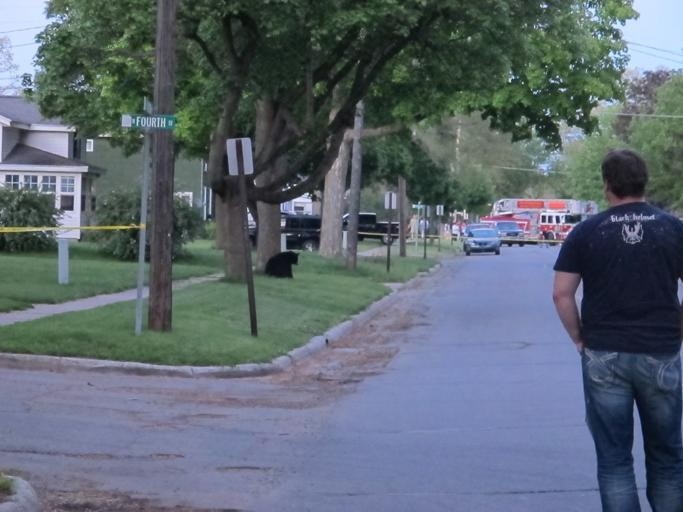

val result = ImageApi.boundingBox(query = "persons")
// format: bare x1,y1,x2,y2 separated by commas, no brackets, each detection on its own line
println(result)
419,219,429,238
445,220,466,242
553,149,682,512
408,213,419,240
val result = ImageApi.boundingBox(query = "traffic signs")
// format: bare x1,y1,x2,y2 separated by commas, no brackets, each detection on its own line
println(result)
121,114,176,129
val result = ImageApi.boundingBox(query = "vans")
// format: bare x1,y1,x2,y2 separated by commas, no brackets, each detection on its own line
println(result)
247,213,321,253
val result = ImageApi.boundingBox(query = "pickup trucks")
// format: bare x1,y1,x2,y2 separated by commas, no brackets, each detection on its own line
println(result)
342,212,399,245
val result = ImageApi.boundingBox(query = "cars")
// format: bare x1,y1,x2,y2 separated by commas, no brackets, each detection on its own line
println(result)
411,217,524,257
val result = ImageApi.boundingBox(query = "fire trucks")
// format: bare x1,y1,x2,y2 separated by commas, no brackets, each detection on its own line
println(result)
480,212,588,247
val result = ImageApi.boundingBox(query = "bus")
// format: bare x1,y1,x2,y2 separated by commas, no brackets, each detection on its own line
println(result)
491,199,597,248
